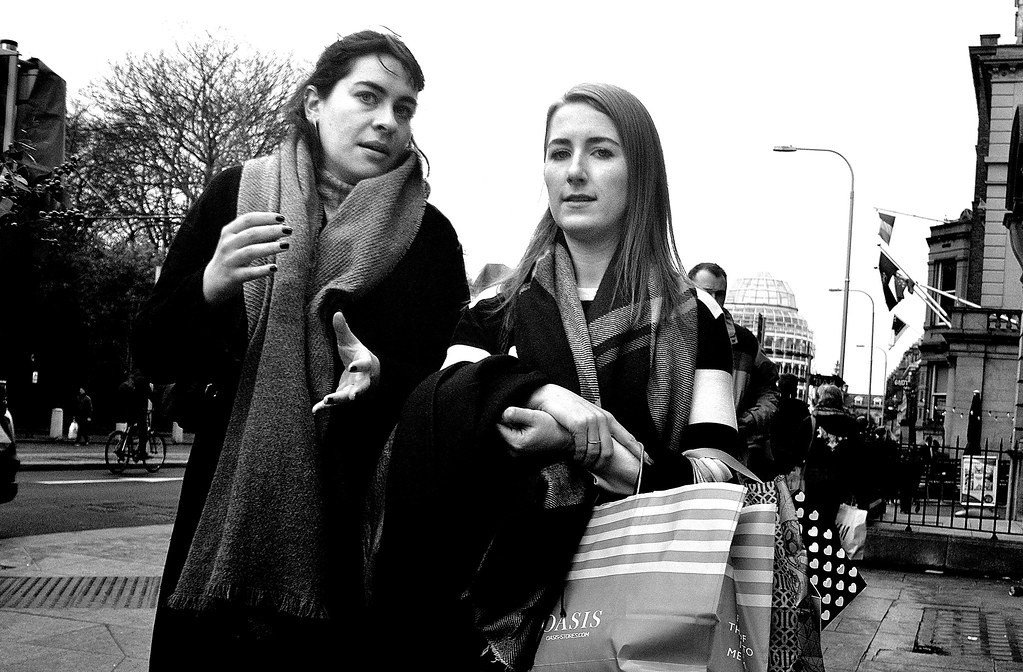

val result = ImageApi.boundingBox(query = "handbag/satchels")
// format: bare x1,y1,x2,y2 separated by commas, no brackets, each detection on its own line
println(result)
68,418,78,439
531,441,868,672
834,496,867,560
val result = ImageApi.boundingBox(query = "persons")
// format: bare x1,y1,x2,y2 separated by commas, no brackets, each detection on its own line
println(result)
685,263,783,468
409,81,738,672
126,375,155,459
75,386,93,445
131,31,471,672
772,371,897,523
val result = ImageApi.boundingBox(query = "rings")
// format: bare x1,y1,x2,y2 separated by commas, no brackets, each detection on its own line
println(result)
587,439,601,445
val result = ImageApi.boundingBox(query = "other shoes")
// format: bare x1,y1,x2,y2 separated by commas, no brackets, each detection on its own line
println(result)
137,453,150,460
114,450,124,460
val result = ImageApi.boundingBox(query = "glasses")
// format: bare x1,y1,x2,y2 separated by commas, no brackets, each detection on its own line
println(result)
705,289,726,299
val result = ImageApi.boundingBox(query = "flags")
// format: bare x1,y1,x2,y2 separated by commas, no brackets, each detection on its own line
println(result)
877,254,899,311
887,316,909,351
905,278,915,294
878,212,895,243
893,272,908,302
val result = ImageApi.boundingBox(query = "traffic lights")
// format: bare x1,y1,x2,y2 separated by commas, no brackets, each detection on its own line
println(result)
15,57,67,182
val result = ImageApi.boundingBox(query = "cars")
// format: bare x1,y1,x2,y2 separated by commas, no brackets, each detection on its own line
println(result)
0,380,20,504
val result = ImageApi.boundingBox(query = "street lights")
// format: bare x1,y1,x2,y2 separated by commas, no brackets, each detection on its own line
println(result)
856,345,887,426
773,146,854,379
829,289,874,428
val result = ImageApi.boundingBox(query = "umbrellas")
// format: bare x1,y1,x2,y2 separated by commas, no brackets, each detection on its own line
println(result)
962,389,983,456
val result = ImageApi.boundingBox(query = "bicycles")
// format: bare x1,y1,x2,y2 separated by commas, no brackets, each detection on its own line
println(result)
105,408,166,474
